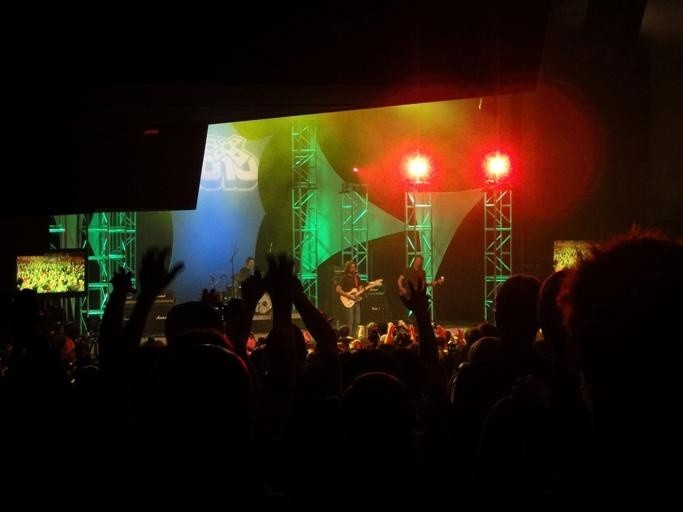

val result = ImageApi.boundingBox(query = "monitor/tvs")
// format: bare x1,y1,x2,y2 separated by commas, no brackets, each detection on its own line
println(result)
12,248,88,298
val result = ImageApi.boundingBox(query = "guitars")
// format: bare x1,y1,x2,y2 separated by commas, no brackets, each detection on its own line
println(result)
340,279,384,309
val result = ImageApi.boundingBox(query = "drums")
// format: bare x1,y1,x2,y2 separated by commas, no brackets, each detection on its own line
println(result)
254,293,271,315
227,286,242,299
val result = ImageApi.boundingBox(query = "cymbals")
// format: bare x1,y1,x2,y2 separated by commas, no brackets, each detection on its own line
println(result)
230,273,251,279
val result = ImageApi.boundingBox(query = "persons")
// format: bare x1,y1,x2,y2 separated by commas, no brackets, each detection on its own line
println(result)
0,222,683,512
